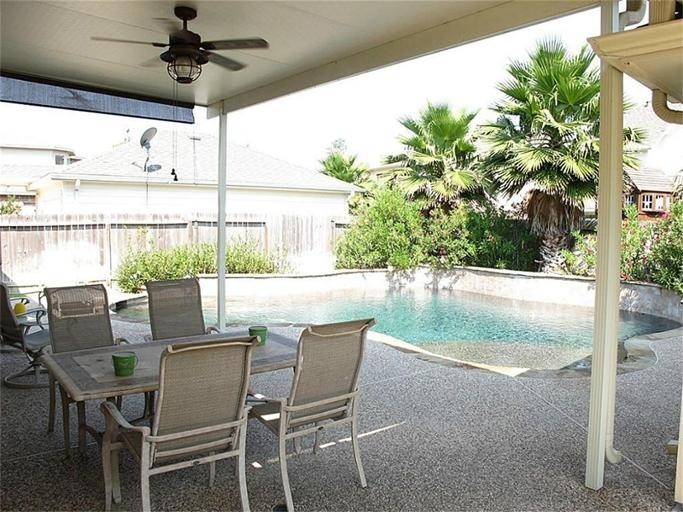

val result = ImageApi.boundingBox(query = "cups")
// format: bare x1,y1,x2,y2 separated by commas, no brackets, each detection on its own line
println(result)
249,326,267,345
111,352,139,376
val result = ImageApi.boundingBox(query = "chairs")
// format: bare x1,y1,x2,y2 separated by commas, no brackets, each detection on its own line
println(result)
144,276,222,347
42,283,131,457
1,280,60,390
231,317,378,512
99,334,261,512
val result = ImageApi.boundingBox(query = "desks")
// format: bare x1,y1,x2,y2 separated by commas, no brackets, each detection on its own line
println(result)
40,327,301,505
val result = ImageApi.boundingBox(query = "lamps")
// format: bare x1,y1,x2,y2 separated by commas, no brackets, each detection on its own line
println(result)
160,4,209,85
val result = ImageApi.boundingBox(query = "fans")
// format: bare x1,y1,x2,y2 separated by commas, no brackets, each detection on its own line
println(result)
90,4,270,72
